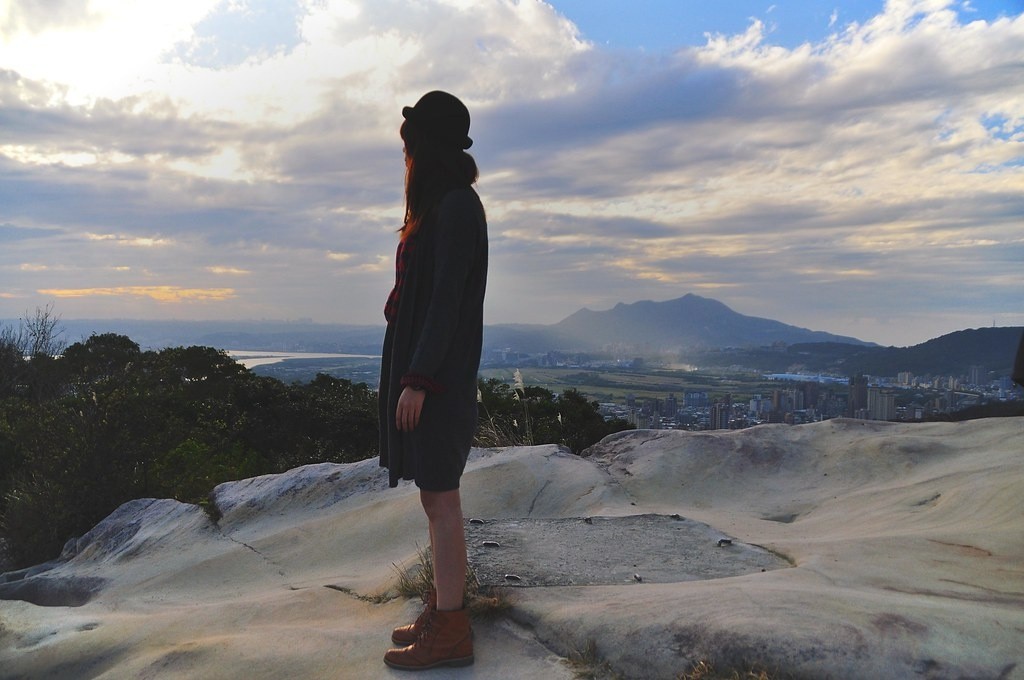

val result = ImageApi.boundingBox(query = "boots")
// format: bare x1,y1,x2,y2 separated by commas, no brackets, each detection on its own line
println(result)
383,588,475,671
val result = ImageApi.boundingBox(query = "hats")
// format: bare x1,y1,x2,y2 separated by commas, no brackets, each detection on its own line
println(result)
402,91,473,149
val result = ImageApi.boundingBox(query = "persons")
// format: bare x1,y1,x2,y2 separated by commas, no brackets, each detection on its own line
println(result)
377,91,488,671
